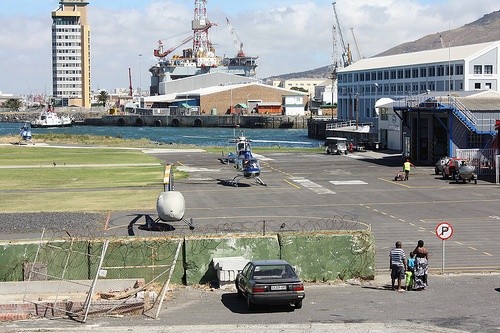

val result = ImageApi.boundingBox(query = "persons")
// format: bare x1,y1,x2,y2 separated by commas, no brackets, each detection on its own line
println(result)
413,240,429,288
407,251,415,269
389,241,407,291
403,159,414,181
404,266,414,291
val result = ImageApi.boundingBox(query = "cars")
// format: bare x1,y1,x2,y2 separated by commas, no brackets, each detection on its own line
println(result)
435,157,478,184
235,260,305,311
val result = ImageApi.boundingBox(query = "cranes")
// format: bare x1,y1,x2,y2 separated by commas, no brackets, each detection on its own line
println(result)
154,0,258,66
331,1,363,77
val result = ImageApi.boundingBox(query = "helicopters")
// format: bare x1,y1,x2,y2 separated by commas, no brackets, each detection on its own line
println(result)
18,120,35,145
219,130,267,187
147,163,195,230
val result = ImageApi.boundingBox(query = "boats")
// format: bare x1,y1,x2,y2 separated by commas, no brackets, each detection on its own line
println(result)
30,104,73,128
